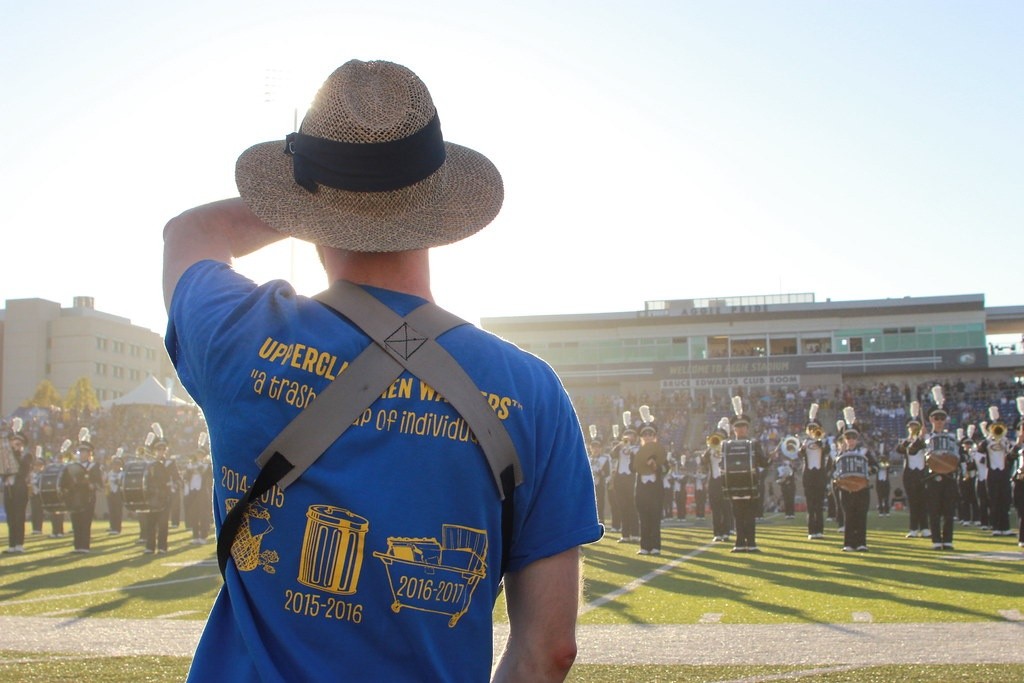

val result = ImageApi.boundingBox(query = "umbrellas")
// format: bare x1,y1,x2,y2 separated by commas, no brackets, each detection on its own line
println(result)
101,375,189,406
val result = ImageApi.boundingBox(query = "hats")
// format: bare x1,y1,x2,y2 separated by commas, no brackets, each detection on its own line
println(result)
730,415,751,426
961,436,974,445
833,433,844,444
621,425,638,436
590,437,602,445
610,437,622,445
841,424,860,434
638,422,657,435
153,438,169,447
714,428,728,440
35,458,45,465
197,446,209,454
1013,416,1024,430
805,418,821,429
925,404,949,421
113,457,125,470
236,61,502,253
905,417,923,428
78,441,94,451
10,430,28,445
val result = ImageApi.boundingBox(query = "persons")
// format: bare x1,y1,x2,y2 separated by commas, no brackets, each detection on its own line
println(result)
577,377,1024,554
711,345,766,372
806,345,820,354
0,404,216,554
162,58,604,683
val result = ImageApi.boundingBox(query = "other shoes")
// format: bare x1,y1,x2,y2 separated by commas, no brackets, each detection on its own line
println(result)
71,548,90,554
637,548,660,555
661,518,686,523
933,543,953,551
144,550,153,554
982,525,993,530
610,528,622,533
32,531,42,534
907,529,932,538
191,537,206,545
958,520,981,527
808,533,823,539
879,514,891,518
843,546,868,551
714,535,730,541
158,549,167,556
838,527,845,533
617,536,639,544
992,530,1016,536
109,531,118,535
730,547,757,552
826,517,833,522
136,538,145,543
785,515,795,519
696,517,705,520
4,545,24,553
170,525,177,528
729,530,735,536
1019,542,1024,547
49,534,63,538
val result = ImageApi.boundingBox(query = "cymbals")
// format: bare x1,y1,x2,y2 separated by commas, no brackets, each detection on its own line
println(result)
632,441,667,476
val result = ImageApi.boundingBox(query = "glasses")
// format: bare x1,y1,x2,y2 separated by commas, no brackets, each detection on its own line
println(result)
845,434,857,440
933,415,947,421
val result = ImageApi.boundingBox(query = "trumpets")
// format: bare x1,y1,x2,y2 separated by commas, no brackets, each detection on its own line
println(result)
704,431,725,451
808,425,827,442
990,421,1009,442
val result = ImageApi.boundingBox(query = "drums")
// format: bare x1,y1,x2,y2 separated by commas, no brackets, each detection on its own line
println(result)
36,450,156,511
923,432,965,475
831,451,871,495
718,436,763,512
